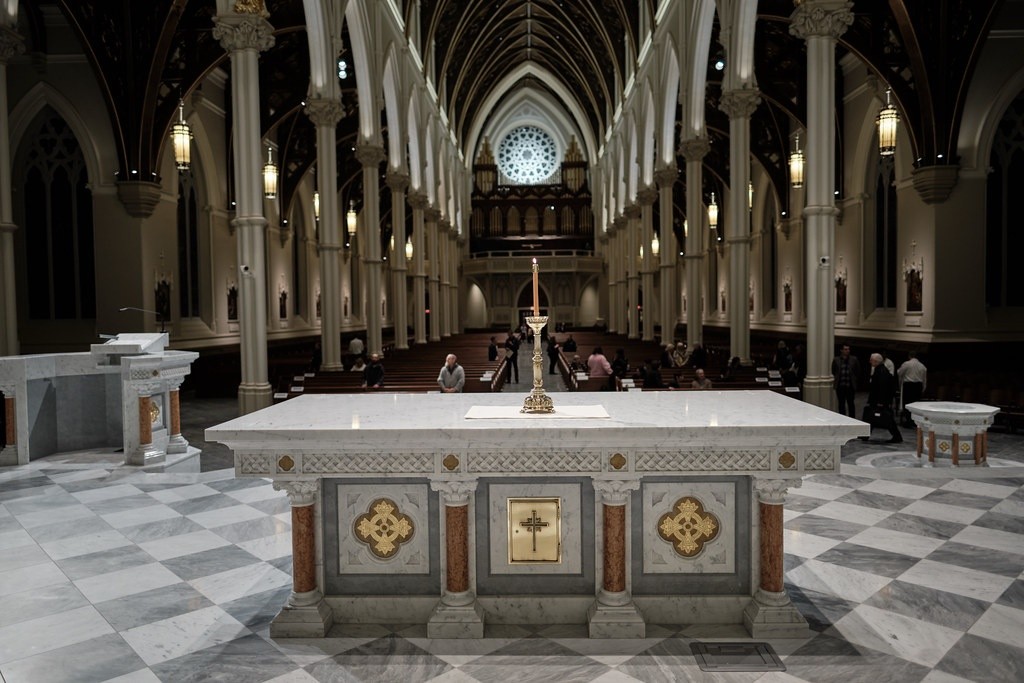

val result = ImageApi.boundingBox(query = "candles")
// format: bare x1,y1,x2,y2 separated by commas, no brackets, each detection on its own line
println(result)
532,257,539,316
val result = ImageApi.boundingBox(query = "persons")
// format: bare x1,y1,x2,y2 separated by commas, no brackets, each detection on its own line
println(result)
546,336,560,376
570,346,630,391
351,353,385,388
437,354,465,392
563,334,577,352
520,323,547,345
773,340,807,401
633,342,746,390
488,336,499,361
831,342,862,419
856,351,926,444
505,330,520,385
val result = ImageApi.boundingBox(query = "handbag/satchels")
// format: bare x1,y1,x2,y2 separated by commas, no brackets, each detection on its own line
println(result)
863,401,894,429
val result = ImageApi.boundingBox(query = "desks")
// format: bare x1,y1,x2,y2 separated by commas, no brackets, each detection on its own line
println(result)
906,402,1000,466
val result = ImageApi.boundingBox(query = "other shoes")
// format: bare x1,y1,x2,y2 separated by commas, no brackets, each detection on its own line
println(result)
857,437,869,441
549,373,556,374
506,382,511,384
516,381,518,383
885,436,903,443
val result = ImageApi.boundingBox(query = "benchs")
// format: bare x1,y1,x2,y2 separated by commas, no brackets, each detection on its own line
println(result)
547,330,799,399
273,333,521,393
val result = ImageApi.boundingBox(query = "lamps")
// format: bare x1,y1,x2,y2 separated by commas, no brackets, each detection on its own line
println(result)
406,235,414,262
347,200,357,237
875,87,899,156
707,191,718,229
639,244,644,260
651,231,659,256
683,219,688,236
749,181,753,212
788,132,805,189
313,192,319,222
262,132,279,199
167,100,195,170
